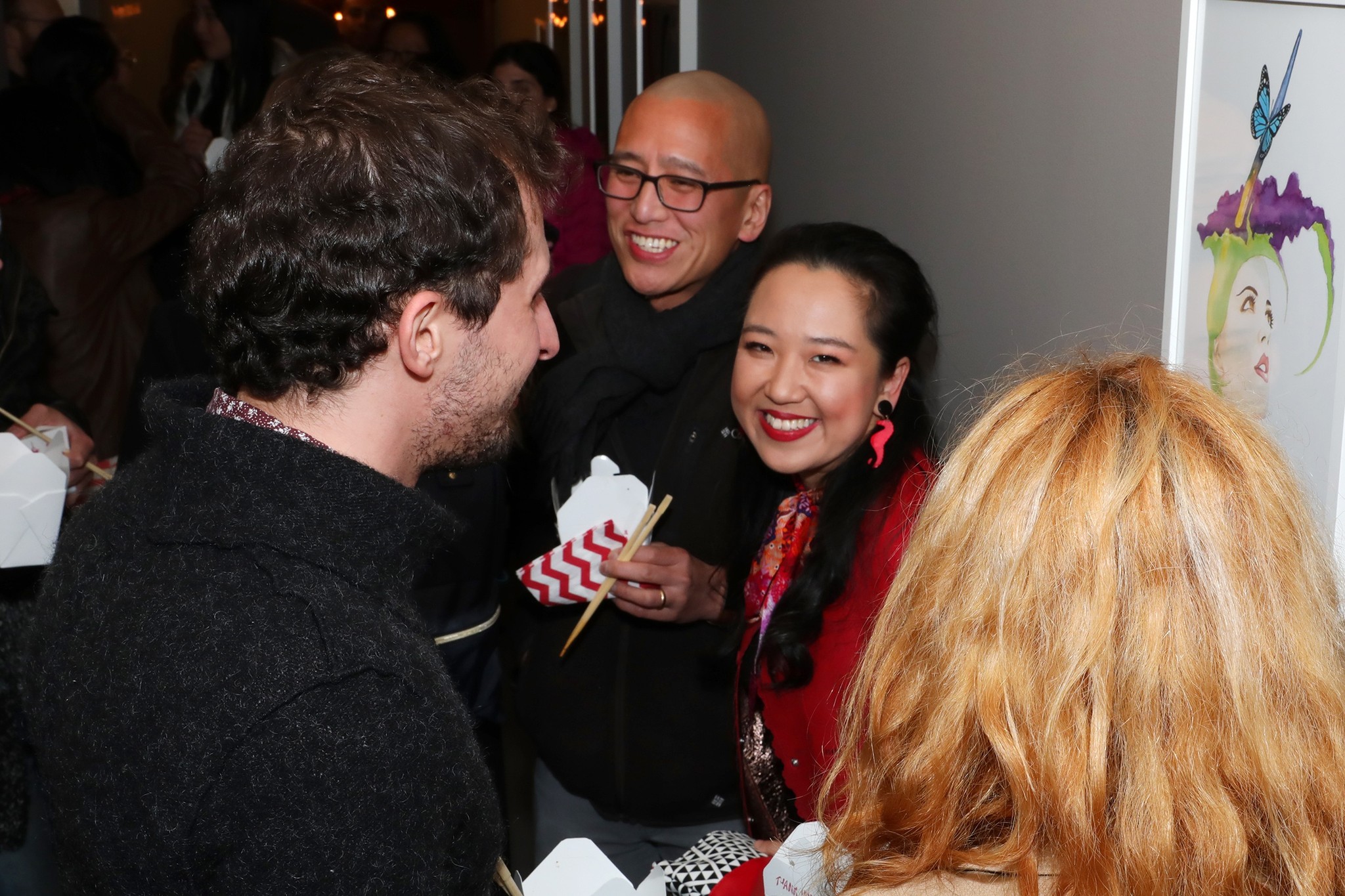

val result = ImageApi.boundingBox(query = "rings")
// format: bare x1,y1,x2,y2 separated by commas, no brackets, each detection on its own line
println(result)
660,589,666,607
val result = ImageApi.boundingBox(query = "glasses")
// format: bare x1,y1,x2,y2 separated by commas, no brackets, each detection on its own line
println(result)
382,48,423,65
593,158,763,214
117,50,138,68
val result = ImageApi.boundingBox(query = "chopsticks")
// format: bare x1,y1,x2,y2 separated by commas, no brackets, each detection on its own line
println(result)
560,493,673,657
494,855,522,896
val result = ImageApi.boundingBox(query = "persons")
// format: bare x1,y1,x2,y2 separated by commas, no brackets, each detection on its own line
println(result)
0,220,94,603
481,41,610,276
721,222,939,842
0,0,25,76
0,15,144,196
157,0,273,162
327,0,417,68
0,91,209,458
412,68,773,834
0,47,561,896
376,13,446,69
711,344,1345,896
